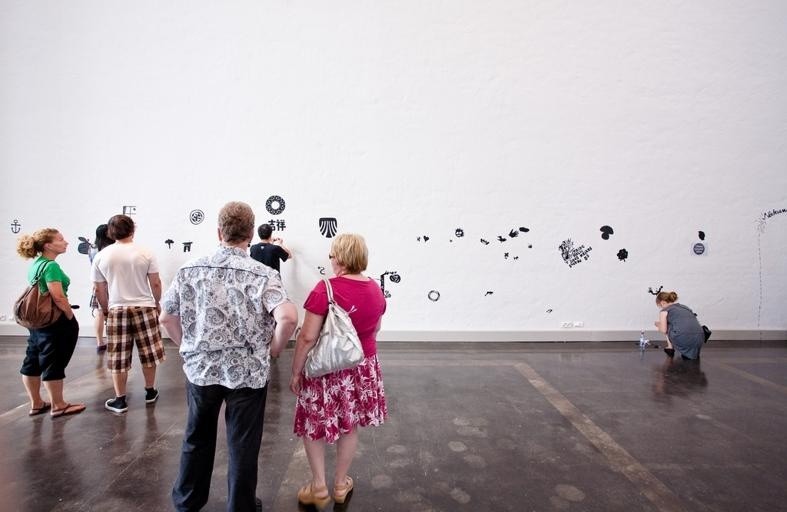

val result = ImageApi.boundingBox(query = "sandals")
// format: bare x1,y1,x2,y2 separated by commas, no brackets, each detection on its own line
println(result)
330,475,353,504
296,481,334,512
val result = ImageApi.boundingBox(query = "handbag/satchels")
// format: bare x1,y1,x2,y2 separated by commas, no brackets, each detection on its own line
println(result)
13,282,63,330
303,303,366,380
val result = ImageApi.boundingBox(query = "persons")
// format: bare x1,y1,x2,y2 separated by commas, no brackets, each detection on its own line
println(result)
288,233,387,510
87,224,114,354
653,290,704,359
156,200,298,511
90,214,162,414
18,227,86,417
251,223,291,317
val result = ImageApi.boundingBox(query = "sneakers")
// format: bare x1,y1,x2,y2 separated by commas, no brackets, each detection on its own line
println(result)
104,398,128,412
28,399,51,415
50,401,86,417
144,387,159,403
96,340,107,352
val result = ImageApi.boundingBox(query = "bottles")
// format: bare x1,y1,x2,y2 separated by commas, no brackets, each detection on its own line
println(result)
640,331,646,350
640,351,646,365
